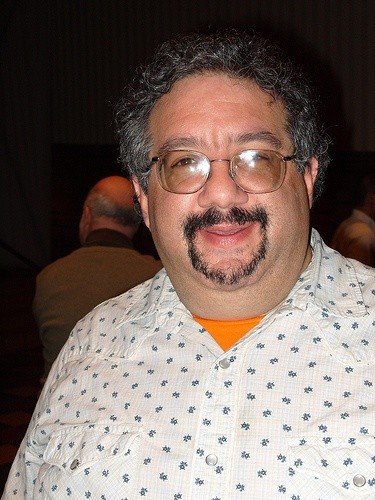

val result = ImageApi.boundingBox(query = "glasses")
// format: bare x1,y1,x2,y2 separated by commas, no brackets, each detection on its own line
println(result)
149,148,294,195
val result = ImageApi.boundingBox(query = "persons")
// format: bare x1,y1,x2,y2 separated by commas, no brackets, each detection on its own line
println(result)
29,176,164,384
0,40,375,499
329,166,375,270
